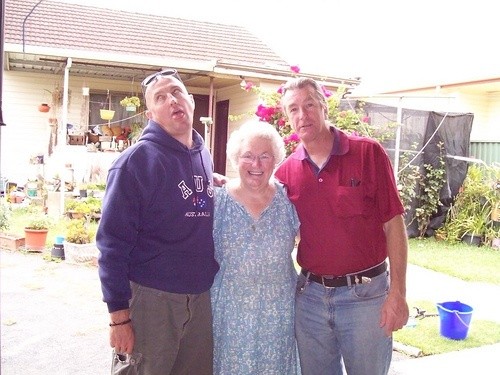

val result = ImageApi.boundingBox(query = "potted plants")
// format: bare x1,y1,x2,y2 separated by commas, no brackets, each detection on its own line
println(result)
0,161,112,263
434,159,500,250
120,95,142,112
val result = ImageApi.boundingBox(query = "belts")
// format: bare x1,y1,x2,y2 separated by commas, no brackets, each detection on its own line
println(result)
301,262,386,289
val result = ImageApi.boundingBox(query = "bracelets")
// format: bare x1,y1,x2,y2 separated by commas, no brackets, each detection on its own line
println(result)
109,319,131,327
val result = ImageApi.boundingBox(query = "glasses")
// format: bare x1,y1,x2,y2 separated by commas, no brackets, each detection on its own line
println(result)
239,152,274,166
141,69,182,96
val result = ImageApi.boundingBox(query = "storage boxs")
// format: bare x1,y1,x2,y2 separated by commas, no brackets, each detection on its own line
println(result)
100,108,115,120
68,134,85,145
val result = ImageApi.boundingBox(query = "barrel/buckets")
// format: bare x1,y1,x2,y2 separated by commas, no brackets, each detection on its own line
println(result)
100,95,115,120
436,301,473,341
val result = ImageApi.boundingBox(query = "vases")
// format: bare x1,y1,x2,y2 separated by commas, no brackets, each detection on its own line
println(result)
37,103,51,113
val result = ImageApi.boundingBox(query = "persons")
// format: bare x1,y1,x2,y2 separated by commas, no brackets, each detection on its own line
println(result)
212,76,409,375
96,76,219,375
210,121,301,375
